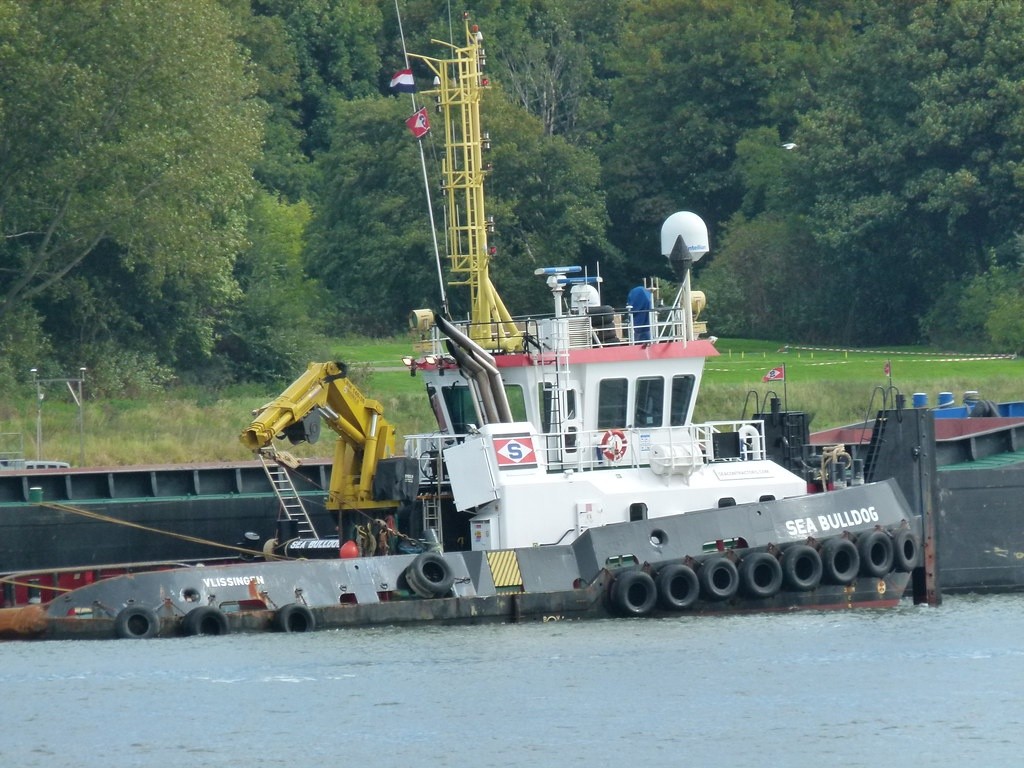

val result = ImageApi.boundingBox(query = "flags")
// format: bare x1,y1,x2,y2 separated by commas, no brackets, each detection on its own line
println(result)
762,365,786,383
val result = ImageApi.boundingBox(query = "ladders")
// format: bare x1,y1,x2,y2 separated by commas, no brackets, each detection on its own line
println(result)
256,441,320,542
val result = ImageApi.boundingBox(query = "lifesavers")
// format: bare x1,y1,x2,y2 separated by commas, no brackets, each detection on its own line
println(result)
182,606,230,637
893,532,919,572
609,571,657,617
397,552,454,598
856,529,895,577
820,537,861,585
781,545,822,590
654,563,701,611
740,552,783,598
116,605,161,638
276,603,314,633
699,558,740,599
602,431,627,460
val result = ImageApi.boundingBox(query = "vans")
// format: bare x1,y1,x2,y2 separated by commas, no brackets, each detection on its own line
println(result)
23,461,71,470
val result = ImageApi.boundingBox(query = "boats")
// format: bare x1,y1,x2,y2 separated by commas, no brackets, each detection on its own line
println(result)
0,390,1023,594
0,0,922,643
